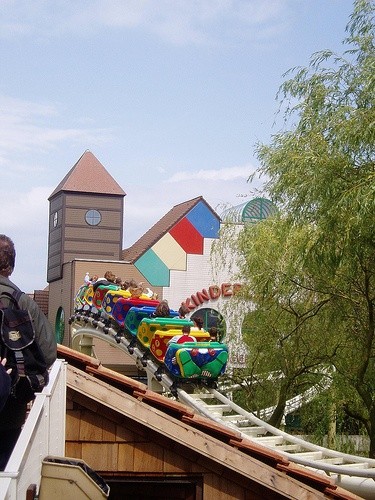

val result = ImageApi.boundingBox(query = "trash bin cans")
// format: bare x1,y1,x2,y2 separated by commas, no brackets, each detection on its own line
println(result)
37,456,111,500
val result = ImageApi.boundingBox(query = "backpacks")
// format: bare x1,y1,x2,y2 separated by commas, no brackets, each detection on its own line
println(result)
0,290,49,402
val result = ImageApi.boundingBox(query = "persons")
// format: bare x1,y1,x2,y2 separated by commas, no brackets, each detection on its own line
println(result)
148,293,159,302
84,271,153,298
127,289,142,302
166,325,197,347
0,234,57,471
149,302,170,319
200,327,222,344
172,307,189,319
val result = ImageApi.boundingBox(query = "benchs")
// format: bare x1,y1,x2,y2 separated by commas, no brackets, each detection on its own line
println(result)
164,339,229,379
114,298,161,324
124,307,181,334
91,284,119,307
149,329,209,362
137,318,195,347
101,291,153,316
